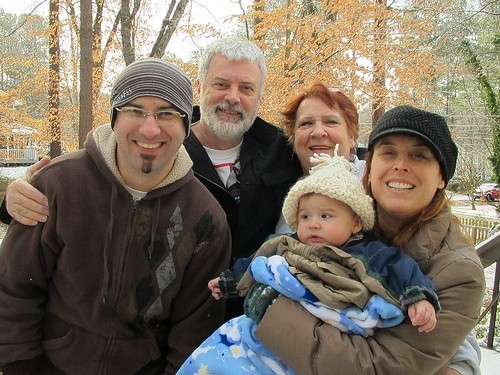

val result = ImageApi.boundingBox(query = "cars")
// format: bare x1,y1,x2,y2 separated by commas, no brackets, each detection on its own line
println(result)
474,184,500,200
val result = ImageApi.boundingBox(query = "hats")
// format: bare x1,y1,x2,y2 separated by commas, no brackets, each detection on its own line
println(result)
283,156,375,232
110,58,192,138
369,106,458,187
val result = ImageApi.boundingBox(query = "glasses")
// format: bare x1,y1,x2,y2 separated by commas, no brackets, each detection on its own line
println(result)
115,107,186,123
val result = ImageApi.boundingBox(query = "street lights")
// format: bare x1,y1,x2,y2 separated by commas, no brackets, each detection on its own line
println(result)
13,101,24,120
